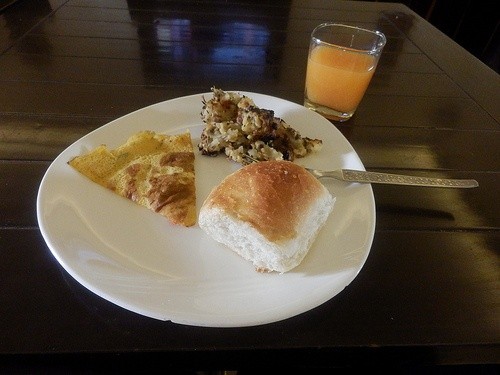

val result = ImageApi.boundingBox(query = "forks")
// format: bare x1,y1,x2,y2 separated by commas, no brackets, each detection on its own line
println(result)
238,151,480,190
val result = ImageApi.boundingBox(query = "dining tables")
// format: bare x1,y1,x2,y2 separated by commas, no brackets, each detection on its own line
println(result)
0,0,500,375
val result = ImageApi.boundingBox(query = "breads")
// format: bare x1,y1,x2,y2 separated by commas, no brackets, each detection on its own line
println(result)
196,160,337,275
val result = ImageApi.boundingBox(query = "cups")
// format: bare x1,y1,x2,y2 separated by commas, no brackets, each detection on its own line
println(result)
302,22,387,126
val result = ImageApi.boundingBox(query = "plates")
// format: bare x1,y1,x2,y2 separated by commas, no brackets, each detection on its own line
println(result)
37,90,377,328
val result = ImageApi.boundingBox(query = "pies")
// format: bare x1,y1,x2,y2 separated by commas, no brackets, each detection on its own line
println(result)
67,130,196,230
